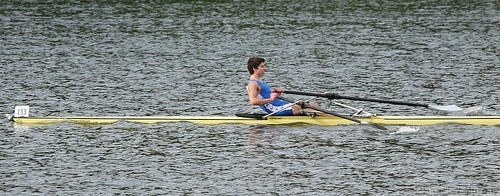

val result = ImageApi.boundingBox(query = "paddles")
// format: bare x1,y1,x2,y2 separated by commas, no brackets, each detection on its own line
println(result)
276,96,388,130
275,89,463,112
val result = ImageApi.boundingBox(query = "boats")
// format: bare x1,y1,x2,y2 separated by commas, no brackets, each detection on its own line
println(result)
12,88,499,130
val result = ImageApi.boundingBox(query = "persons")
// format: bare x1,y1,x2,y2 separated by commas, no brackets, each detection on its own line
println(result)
246,57,333,116
